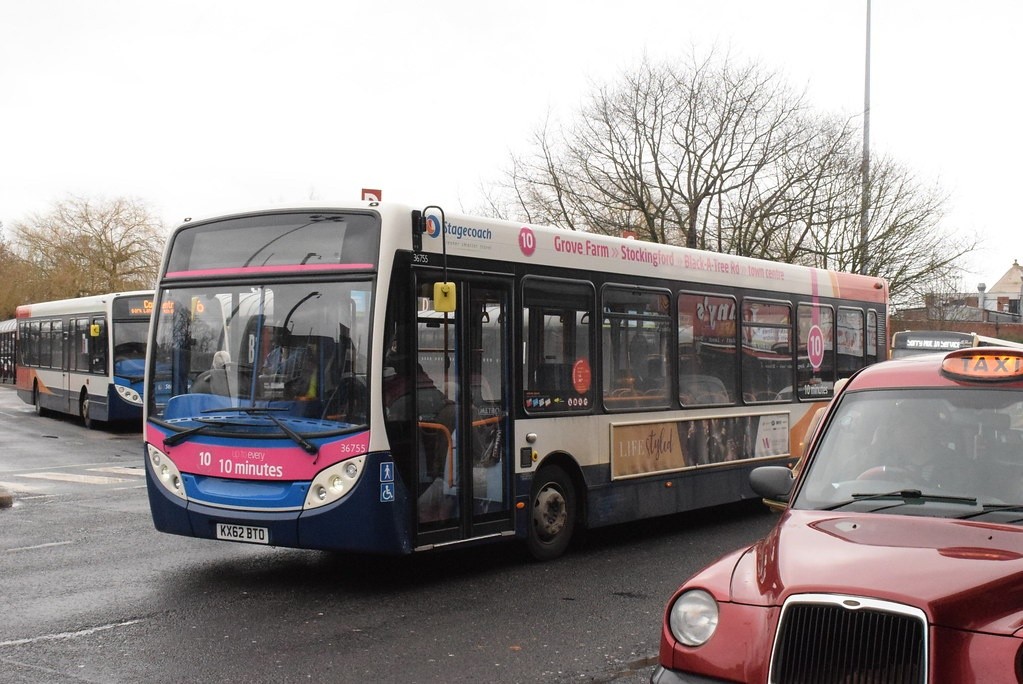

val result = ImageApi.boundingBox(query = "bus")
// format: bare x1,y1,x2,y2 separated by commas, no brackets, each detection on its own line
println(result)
0,319,17,385
16,290,224,430
891,329,1023,360
146,202,891,563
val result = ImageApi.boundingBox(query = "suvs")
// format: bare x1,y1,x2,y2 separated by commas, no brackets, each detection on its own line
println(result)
648,345,1023,684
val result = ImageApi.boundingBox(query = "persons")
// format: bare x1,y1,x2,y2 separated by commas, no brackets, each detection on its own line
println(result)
440,428,456,519
262,321,315,396
383,354,445,420
